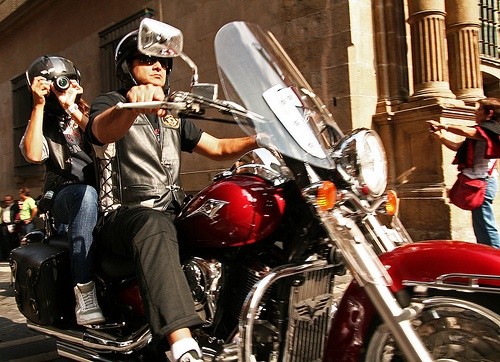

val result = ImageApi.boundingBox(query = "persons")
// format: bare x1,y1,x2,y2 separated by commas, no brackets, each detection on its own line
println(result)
19,52,117,325
427,98,500,248
84,29,273,362
0,187,37,262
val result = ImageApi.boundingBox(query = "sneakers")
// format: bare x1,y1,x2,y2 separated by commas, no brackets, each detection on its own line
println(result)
74,280,106,325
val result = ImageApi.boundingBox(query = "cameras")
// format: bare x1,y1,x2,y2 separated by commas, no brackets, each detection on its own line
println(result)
53,76,71,92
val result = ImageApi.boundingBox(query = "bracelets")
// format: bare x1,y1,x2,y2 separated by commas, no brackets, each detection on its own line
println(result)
66,104,78,114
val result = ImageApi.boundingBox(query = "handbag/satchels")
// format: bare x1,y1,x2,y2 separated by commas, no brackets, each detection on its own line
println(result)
447,173,487,210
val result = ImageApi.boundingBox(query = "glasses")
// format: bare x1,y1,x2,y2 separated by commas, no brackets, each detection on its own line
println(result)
129,53,169,71
476,107,486,113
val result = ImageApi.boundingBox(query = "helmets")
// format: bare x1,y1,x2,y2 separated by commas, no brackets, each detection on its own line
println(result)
26,56,81,93
115,28,173,75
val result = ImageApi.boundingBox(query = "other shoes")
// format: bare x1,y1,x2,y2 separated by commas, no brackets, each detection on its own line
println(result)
164,339,207,362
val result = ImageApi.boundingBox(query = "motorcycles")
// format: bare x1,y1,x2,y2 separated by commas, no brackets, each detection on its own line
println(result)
9,20,500,361
